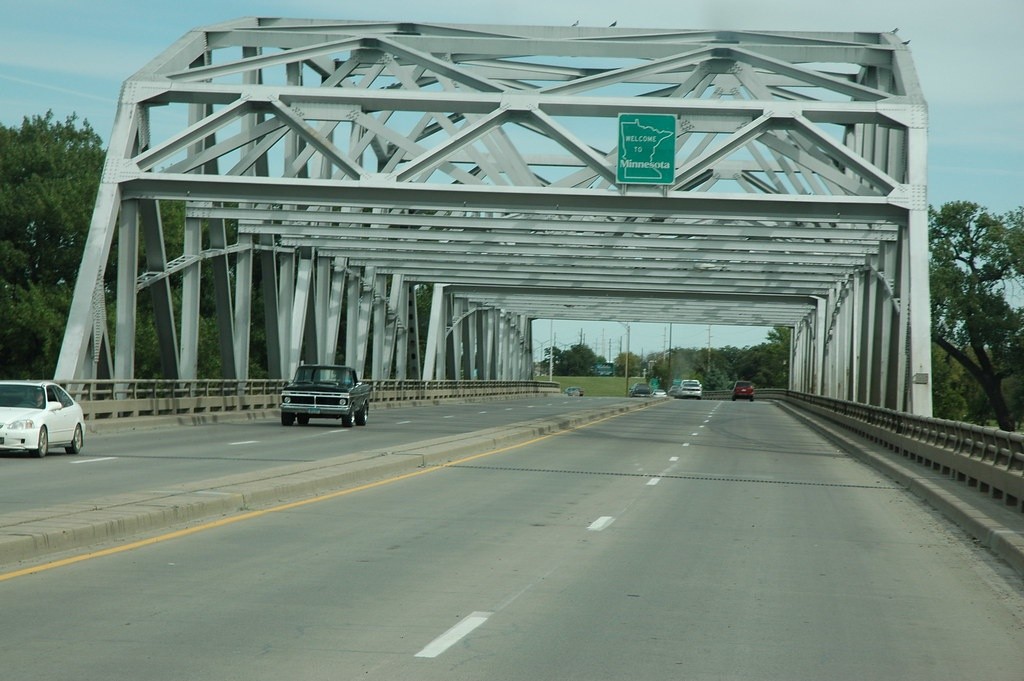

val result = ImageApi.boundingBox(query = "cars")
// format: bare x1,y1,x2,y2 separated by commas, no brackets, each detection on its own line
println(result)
667,379,703,400
629,382,650,397
565,387,584,397
0,382,87,457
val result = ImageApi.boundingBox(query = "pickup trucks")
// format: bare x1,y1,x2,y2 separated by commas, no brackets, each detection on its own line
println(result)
280,364,371,428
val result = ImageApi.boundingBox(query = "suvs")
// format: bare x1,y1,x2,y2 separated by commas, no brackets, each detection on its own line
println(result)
732,381,755,402
